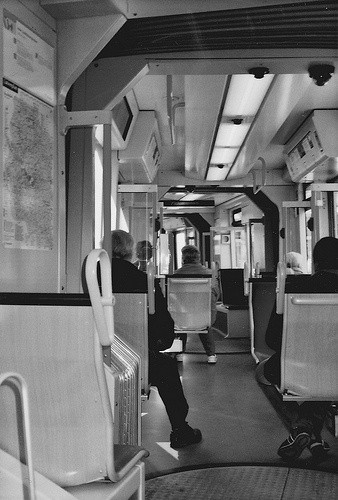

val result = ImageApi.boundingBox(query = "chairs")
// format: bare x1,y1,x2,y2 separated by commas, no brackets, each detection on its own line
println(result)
0,269,338,487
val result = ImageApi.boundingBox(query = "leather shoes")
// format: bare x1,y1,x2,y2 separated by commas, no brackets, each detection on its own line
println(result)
170,426,202,448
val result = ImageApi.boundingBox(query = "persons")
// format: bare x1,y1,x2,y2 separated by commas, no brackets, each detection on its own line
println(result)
255,236,338,459
175,245,218,363
97,229,202,449
132,240,158,275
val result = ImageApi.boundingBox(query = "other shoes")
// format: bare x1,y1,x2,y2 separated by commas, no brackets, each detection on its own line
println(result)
207,354,217,363
176,354,183,362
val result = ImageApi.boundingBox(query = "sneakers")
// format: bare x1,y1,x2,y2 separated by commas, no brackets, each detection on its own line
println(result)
278,428,312,459
307,437,330,456
